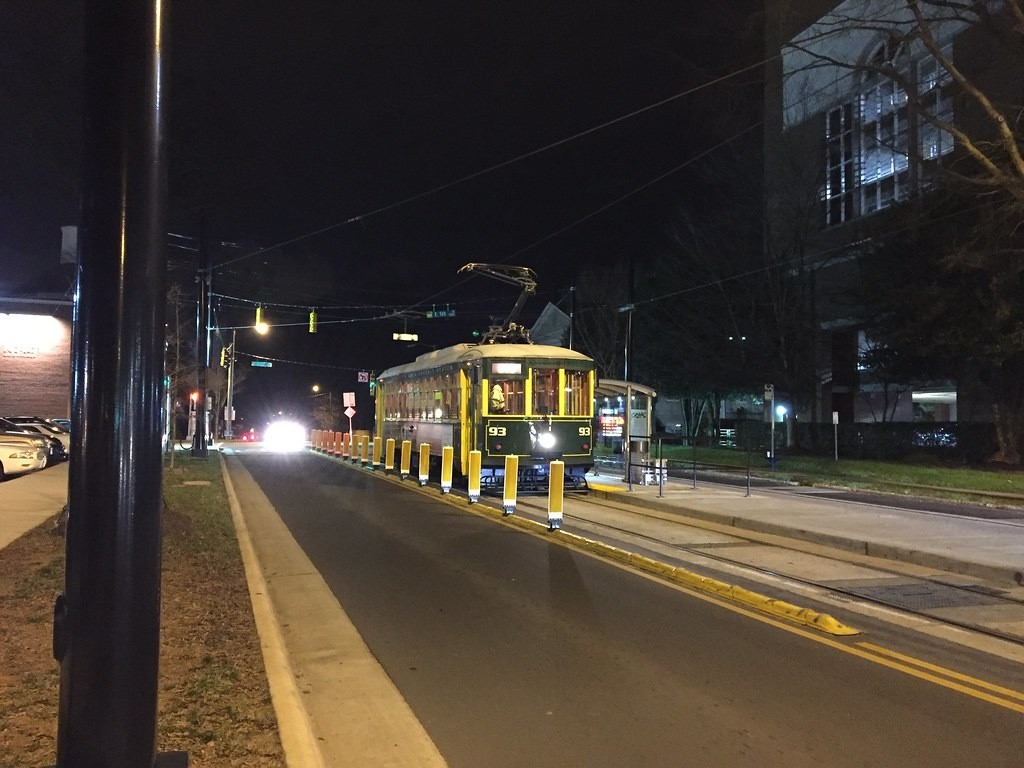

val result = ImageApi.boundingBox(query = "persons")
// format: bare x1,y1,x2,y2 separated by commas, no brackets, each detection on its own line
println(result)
490,383,506,416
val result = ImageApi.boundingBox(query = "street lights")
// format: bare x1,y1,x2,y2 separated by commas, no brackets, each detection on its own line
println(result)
616,303,634,483
312,385,333,411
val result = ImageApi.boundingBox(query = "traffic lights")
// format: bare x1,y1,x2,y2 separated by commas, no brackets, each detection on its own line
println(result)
369,373,375,396
219,347,231,369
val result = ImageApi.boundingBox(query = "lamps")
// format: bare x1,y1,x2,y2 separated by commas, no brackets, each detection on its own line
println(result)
308,308,318,332
253,303,270,335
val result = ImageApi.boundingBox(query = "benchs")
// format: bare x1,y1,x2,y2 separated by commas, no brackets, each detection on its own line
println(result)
593,455,627,476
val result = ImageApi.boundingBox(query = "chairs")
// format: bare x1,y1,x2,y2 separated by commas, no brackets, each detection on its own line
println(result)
537,406,556,415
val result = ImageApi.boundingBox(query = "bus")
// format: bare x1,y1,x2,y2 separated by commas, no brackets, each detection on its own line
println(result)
372,340,599,493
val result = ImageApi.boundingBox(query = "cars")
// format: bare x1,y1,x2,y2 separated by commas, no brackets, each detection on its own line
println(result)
0,414,71,477
